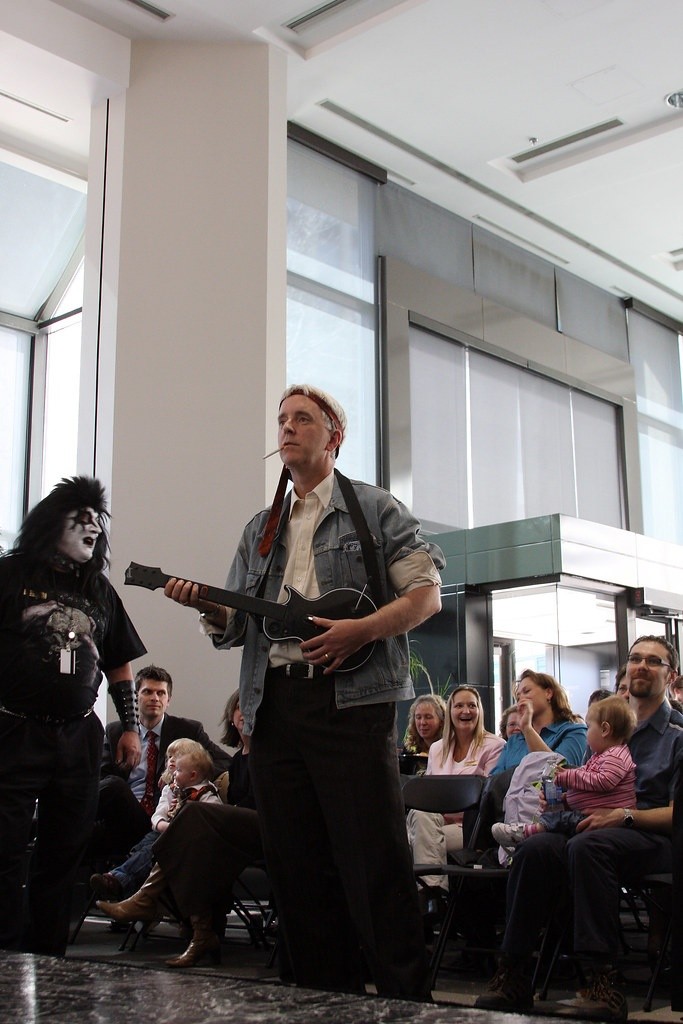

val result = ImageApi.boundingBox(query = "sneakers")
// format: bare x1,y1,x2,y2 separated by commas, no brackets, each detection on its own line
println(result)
491,822,525,847
474,968,534,1011
577,965,628,1024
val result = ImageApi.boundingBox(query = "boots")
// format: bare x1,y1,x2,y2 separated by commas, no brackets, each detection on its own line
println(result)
165,912,221,968
96,862,168,939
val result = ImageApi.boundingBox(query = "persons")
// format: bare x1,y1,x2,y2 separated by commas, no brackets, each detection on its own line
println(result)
88,631,683,1019
204,384,443,1006
0,475,148,957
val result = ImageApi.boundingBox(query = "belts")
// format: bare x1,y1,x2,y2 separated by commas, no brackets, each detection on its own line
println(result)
275,663,313,679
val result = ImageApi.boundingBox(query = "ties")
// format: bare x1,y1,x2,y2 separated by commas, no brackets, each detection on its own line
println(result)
139,731,159,817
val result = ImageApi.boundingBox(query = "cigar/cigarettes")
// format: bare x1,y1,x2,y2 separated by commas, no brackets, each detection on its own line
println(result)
262,445,283,460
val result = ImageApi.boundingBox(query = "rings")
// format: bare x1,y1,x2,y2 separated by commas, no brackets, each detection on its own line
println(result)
325,654,330,661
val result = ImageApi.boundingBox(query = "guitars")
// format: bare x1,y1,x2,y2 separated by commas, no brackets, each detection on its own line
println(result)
123,560,378,673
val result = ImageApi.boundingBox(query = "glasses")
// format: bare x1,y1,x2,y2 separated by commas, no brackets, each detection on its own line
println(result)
617,686,627,695
626,654,670,667
507,722,519,727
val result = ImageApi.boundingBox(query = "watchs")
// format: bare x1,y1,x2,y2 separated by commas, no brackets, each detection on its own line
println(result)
623,808,635,827
199,604,220,620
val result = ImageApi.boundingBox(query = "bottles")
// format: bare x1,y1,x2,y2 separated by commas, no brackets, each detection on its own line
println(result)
540,756,565,814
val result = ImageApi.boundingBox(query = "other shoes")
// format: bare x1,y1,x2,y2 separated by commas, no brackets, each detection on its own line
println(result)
421,894,445,924
90,873,122,900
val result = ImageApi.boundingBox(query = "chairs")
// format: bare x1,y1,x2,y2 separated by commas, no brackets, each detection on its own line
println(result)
67,763,683,1013
